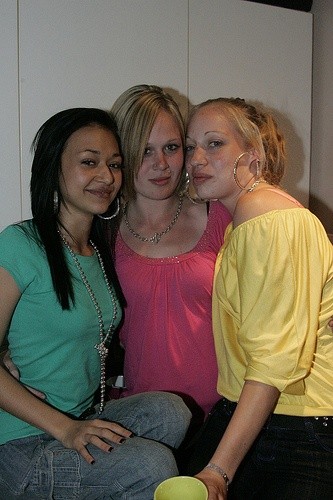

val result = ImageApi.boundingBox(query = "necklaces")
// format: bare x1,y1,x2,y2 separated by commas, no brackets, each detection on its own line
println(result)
124,191,183,242
56,225,116,418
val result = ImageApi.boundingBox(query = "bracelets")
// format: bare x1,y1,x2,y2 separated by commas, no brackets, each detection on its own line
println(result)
205,461,231,488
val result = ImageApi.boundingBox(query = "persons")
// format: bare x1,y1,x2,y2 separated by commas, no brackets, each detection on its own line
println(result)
109,83,234,464
1,107,192,499
186,97,333,499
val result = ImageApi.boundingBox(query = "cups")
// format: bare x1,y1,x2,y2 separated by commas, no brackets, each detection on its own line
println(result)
153,476,209,500
